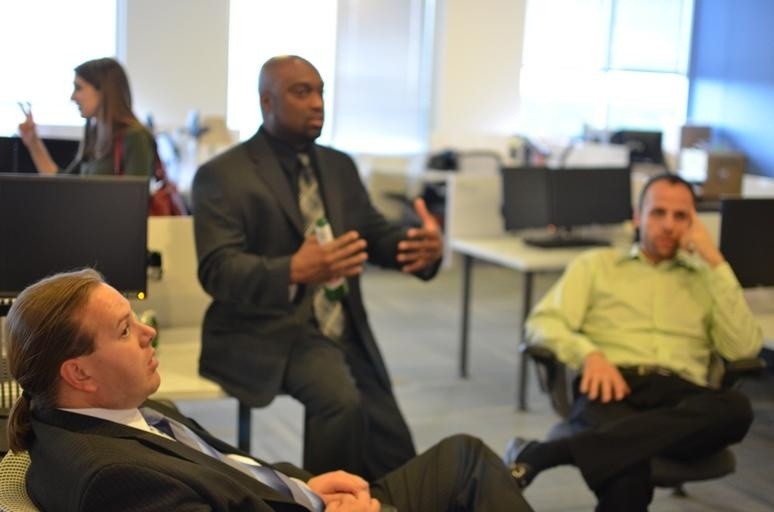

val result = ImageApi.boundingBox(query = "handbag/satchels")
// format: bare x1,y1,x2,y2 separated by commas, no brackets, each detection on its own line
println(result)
111,121,188,215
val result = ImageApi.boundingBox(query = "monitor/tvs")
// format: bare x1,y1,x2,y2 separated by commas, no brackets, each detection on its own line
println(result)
500,163,632,249
1,134,79,173
719,197,774,288
611,131,662,164
0,173,152,301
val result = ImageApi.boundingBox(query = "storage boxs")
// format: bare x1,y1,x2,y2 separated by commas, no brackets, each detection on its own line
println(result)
677,124,748,198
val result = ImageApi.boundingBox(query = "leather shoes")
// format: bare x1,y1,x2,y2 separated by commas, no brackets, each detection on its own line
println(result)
502,437,538,494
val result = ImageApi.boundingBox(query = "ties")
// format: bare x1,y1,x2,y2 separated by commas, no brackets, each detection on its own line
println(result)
141,406,326,511
297,150,345,343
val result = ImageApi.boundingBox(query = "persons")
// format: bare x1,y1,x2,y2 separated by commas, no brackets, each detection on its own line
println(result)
192,57,442,489
16,59,156,178
3,265,540,509
510,174,767,510
